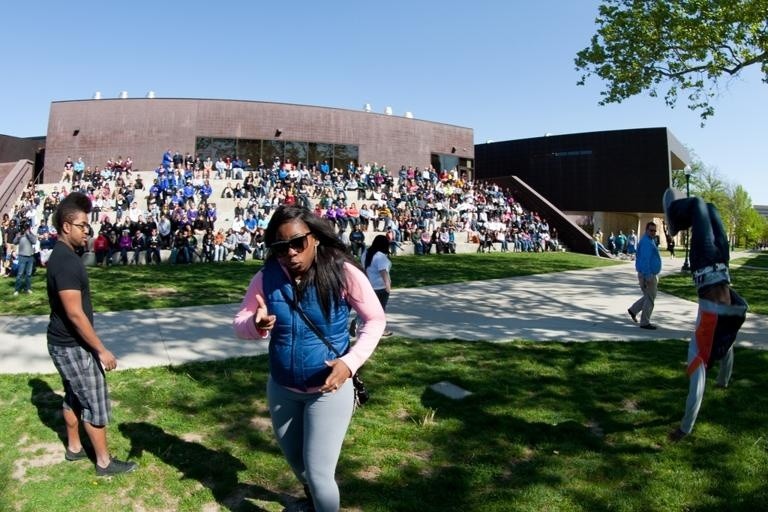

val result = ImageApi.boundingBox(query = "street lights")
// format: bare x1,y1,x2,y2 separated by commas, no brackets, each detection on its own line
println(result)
682,164,695,270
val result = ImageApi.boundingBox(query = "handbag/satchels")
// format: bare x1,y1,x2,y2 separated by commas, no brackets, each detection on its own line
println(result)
353,371,370,406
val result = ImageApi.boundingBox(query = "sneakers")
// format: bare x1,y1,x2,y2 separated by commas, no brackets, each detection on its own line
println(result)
662,188,686,237
13,288,34,296
380,329,395,339
95,454,138,478
64,445,96,462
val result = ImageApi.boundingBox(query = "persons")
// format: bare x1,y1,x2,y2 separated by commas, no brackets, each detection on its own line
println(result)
662,188,749,444
594,227,637,261
45,192,137,474
667,238,675,259
234,205,386,512
628,221,661,330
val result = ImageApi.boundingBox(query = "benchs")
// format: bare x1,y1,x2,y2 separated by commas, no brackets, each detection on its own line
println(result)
22,175,547,268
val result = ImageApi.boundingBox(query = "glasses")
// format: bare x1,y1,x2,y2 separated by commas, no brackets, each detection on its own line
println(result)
70,222,91,230
648,228,657,233
270,231,314,257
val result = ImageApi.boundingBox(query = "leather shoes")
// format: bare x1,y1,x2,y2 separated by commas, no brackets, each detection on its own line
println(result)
628,308,638,323
640,323,656,330
